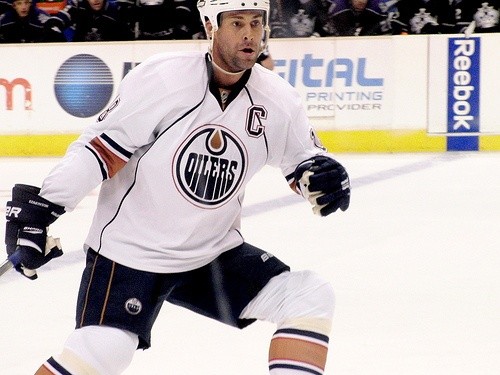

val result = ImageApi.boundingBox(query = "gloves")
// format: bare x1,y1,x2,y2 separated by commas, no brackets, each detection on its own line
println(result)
292,155,351,216
5,183,63,280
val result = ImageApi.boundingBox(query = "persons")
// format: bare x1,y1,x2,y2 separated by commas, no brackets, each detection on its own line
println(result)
269,0,500,37
5,0,351,375
0,0,207,45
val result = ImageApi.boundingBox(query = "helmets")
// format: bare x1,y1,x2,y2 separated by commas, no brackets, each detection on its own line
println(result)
198,0,270,33
